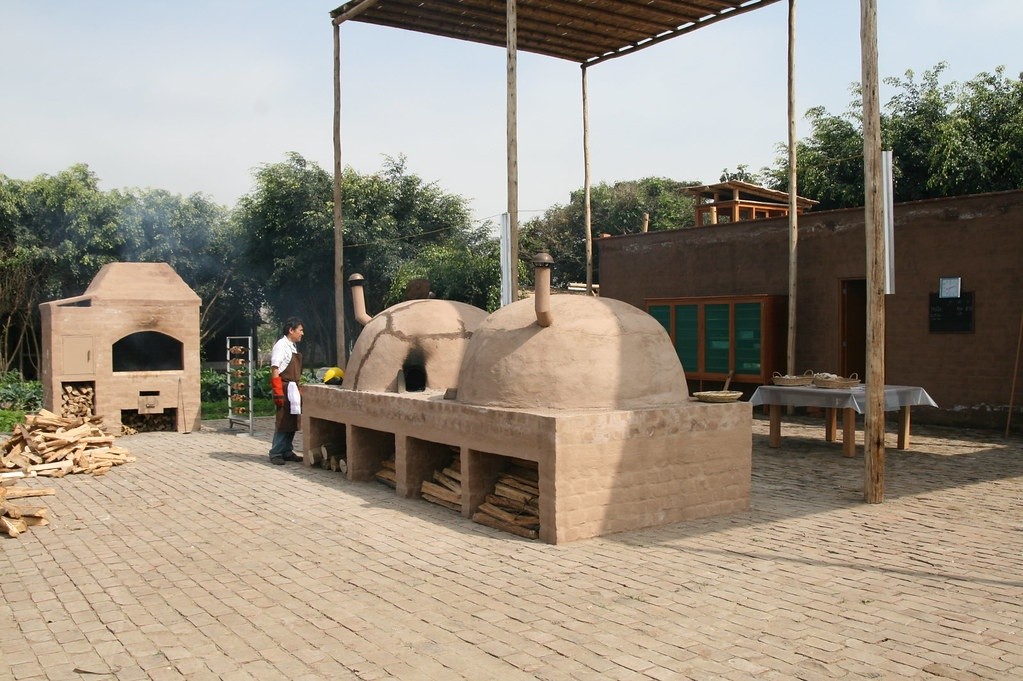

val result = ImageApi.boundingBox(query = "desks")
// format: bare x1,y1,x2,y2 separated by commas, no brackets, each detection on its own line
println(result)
749,382,940,457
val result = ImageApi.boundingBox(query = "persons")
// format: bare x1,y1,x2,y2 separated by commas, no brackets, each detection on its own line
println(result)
269,318,304,466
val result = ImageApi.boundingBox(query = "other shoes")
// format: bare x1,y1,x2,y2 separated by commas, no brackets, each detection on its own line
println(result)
271,458,285,465
284,453,303,462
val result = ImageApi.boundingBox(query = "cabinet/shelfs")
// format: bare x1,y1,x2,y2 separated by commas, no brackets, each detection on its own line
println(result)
643,293,789,416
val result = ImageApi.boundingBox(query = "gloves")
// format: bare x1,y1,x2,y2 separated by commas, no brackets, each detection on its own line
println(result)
270,377,286,406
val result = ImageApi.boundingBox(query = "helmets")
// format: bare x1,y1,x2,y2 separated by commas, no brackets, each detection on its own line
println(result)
324,367,345,386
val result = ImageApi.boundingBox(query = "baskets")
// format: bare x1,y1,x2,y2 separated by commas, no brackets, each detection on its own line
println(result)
814,373,861,388
771,369,817,385
693,390,743,403
